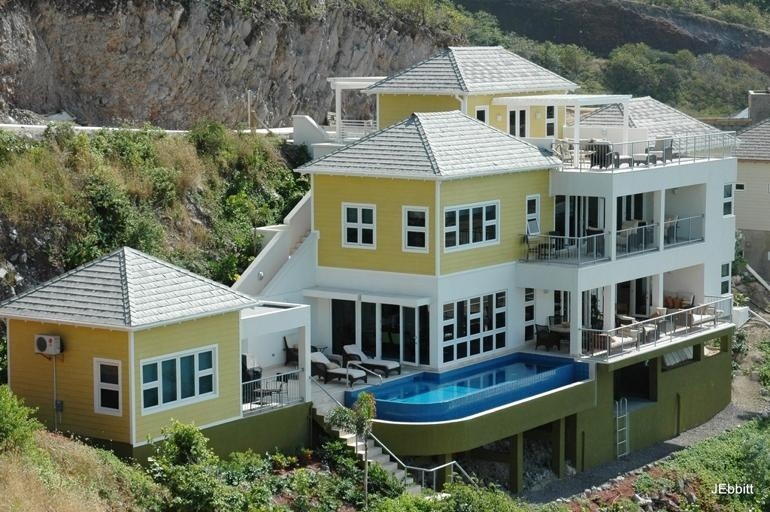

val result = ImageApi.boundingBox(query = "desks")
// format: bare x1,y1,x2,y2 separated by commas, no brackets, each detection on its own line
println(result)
548,322,585,351
311,344,328,353
253,389,272,405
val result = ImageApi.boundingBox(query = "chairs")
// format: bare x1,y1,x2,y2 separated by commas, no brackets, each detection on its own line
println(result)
311,351,367,387
548,315,563,325
585,214,679,257
524,230,569,259
534,323,560,352
590,314,677,355
342,344,401,378
282,336,299,365
271,379,289,404
551,137,674,171
242,353,262,389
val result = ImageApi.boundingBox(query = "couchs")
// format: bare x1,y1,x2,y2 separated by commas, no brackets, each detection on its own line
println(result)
648,290,696,326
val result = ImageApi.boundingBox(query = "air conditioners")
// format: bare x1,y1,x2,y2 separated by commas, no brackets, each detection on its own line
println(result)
34,334,65,355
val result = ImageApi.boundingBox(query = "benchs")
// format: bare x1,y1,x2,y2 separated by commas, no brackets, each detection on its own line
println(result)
672,303,717,333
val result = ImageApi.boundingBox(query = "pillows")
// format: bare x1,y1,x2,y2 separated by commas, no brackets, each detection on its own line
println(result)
663,295,689,309
678,313,694,322
